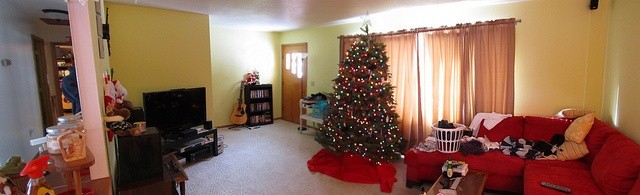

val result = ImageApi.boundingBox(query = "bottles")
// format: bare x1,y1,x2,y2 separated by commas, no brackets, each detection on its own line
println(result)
57,115,73,130
447,156,454,179
46,125,70,155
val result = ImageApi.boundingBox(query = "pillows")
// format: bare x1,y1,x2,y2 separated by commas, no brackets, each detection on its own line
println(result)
561,108,593,118
563,114,595,145
556,141,589,161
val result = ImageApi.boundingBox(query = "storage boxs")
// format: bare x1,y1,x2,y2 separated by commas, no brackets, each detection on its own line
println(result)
311,101,330,119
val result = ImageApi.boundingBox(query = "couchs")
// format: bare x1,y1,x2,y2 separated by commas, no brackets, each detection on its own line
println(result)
403,115,640,195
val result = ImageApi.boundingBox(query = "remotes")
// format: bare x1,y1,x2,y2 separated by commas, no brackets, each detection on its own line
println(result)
541,182,571,193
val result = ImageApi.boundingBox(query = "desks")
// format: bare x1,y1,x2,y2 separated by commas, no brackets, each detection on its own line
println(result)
48,145,96,194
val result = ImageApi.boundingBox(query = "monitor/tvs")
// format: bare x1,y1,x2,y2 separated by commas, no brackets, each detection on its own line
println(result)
143,87,206,140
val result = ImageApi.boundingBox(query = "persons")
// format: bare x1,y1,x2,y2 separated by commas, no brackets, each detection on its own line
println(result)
103,74,131,119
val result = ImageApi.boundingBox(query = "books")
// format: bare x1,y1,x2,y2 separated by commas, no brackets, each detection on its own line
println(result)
249,89,271,99
249,101,271,111
250,111,271,124
441,162,469,176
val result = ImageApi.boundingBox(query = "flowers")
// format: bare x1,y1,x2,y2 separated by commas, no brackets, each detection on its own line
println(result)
243,69,260,84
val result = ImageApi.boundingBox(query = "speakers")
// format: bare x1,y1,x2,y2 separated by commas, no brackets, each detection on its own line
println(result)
203,120,213,130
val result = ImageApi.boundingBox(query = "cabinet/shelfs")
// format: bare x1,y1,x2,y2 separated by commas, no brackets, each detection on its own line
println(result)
115,126,164,190
245,84,274,126
120,153,189,195
166,128,219,164
54,45,74,112
299,98,331,135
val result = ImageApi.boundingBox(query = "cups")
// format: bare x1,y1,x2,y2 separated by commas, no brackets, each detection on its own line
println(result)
134,121,147,132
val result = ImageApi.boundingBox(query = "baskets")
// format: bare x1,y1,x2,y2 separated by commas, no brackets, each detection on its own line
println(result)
431,121,466,154
